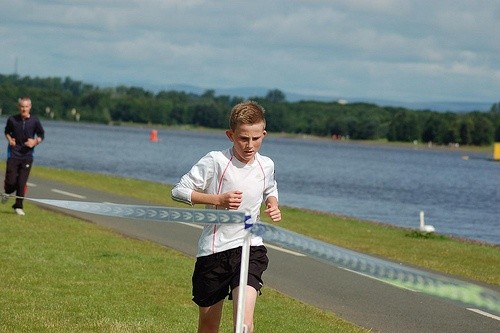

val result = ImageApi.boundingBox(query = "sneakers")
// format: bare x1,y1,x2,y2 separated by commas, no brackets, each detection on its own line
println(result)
15,206,25,217
2,191,13,205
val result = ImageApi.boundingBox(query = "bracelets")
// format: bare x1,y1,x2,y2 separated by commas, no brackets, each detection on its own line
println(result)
35,139,39,145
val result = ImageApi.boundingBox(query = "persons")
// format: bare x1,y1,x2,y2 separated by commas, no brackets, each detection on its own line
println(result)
0,97,45,216
170,100,282,333
